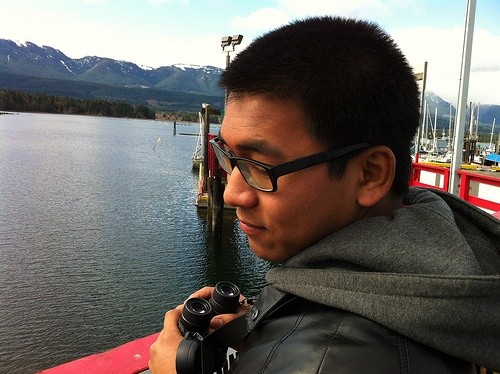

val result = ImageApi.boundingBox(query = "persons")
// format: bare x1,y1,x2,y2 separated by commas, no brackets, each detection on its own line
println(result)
147,15,500,374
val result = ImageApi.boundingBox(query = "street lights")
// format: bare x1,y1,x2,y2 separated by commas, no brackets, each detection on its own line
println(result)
220,34,244,112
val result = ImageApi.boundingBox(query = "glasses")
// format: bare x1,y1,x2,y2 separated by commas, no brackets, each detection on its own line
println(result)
210,136,373,193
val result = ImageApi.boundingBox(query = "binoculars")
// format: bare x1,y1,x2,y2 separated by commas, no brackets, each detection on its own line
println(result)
175,278,243,341
